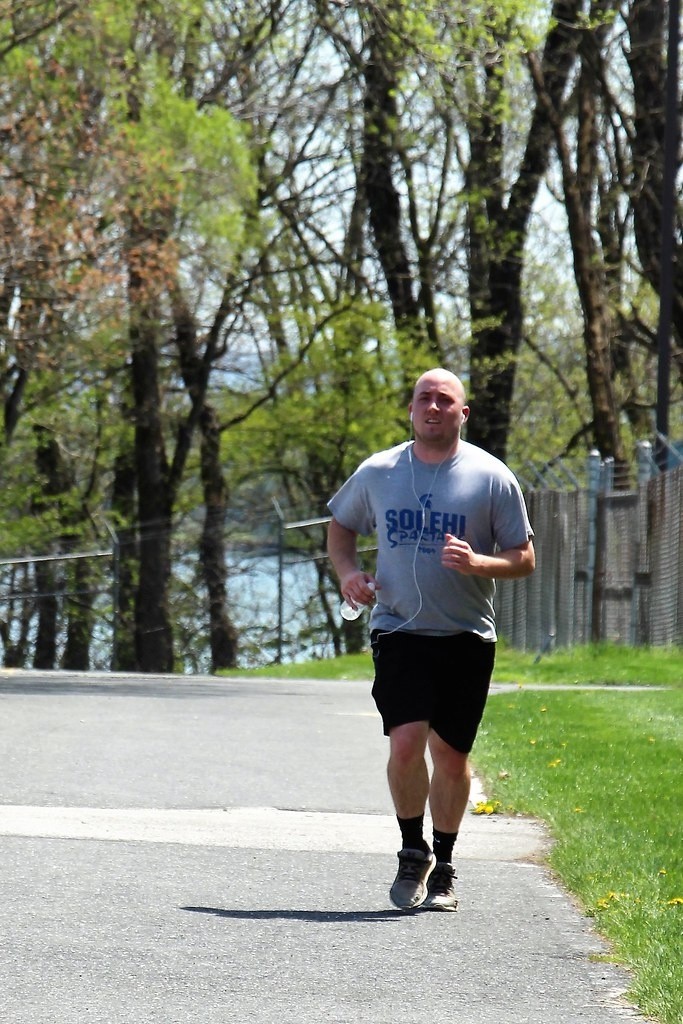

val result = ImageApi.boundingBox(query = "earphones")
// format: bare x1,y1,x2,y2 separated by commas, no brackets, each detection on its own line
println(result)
410,412,413,422
460,414,466,425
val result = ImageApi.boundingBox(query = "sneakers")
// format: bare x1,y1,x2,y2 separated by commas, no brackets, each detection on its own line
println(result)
419,862,459,912
389,841,437,909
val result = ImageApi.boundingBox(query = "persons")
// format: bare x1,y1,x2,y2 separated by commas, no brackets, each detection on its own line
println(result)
325,367,537,911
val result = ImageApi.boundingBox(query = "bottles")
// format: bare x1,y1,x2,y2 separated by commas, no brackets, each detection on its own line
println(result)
340,583,375,620
